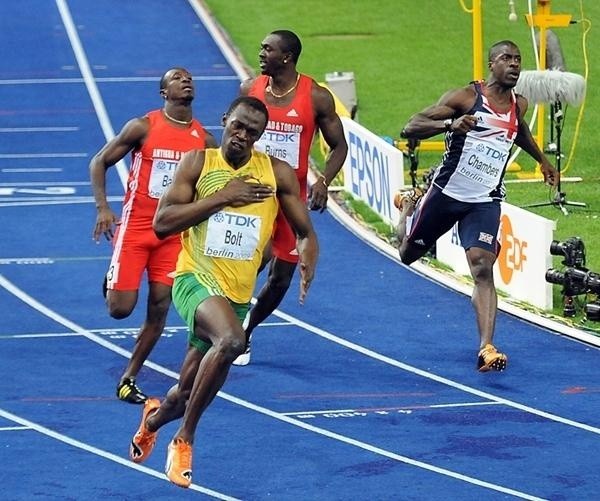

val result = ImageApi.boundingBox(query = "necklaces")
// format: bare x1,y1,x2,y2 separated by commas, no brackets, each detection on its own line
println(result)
163,108,193,125
266,71,301,98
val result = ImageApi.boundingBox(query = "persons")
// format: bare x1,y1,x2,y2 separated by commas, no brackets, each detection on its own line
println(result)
229,29,348,369
393,38,560,375
89,66,217,405
128,94,319,488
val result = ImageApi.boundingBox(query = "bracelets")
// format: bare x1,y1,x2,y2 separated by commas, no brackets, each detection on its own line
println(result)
318,173,330,188
444,117,454,131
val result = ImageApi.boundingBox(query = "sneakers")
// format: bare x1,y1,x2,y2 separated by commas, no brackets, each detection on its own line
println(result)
130,398,161,462
165,438,192,488
478,344,507,372
395,188,423,211
116,378,146,404
232,336,250,365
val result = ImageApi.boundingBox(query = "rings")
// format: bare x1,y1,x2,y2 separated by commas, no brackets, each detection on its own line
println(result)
257,192,259,197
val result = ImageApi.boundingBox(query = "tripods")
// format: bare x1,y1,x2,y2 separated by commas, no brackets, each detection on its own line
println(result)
515,97,587,215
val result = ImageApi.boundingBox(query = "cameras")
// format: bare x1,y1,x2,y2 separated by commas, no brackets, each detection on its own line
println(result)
545,269,579,291
548,237,584,266
